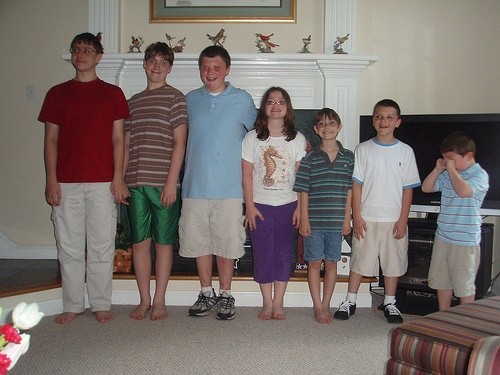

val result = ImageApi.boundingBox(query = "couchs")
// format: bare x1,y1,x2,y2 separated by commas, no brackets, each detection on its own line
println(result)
382,294,500,375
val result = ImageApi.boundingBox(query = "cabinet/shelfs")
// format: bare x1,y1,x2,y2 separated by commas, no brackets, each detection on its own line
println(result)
378,218,494,317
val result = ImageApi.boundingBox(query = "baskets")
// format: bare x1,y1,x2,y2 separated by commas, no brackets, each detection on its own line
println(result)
86,234,132,273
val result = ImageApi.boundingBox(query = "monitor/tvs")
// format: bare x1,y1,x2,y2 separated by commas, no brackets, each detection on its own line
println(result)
359,113,500,216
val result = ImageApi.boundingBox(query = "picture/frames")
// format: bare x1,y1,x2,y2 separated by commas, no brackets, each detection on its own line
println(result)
148,0,297,24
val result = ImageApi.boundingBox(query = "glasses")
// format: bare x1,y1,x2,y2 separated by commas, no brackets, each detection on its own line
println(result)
69,47,97,55
265,99,286,105
318,121,337,128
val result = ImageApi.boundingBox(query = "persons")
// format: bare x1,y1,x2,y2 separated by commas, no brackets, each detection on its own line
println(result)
186,44,256,320
36,33,131,326
241,87,308,319
293,108,356,323
333,99,421,323
422,131,490,311
126,41,189,321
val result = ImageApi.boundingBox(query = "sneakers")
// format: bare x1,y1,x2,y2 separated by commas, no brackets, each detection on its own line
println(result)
216,291,235,320
383,301,403,323
333,300,356,320
189,288,218,317
